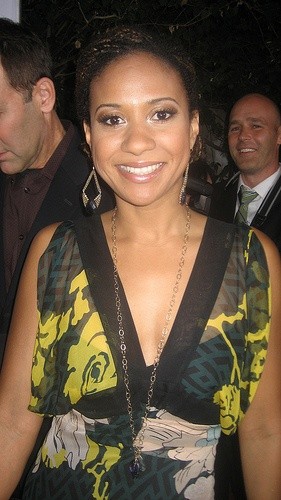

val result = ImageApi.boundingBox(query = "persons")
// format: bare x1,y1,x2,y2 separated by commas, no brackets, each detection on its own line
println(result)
0,28,281,500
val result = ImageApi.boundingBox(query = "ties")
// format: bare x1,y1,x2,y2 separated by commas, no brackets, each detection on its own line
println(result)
233,184,258,226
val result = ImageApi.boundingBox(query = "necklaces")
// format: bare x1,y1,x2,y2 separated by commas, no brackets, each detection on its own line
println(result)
112,204,191,480
